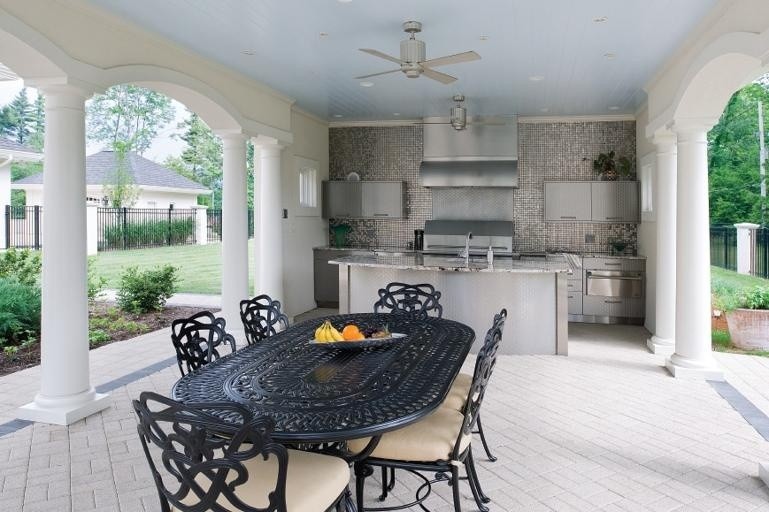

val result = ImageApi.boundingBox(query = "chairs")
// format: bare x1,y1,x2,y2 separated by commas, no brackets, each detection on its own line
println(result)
171,310,237,377
348,328,504,511
432,307,508,464
373,281,442,318
238,294,289,345
133,392,350,512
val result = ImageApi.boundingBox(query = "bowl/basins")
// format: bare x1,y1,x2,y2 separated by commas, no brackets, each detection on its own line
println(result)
610,242,629,252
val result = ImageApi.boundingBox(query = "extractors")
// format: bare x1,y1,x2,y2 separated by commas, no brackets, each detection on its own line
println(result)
419,116,520,190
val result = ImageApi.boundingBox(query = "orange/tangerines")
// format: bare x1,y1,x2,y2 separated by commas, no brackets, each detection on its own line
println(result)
342,325,359,340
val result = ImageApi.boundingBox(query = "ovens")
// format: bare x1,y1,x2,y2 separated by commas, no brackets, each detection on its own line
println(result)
583,256,647,319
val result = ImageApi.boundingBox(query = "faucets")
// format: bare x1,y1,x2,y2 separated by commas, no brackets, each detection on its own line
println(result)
465,232,472,265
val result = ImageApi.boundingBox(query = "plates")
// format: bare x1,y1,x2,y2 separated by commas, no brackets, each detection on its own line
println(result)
310,333,407,350
346,171,360,181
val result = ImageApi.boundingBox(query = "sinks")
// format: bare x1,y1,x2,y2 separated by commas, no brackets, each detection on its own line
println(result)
448,257,484,262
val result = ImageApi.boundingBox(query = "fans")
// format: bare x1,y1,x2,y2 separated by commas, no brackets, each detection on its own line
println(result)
353,19,478,84
412,95,505,131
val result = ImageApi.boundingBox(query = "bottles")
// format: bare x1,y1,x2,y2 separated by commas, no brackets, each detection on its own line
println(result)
407,241,412,249
414,230,423,250
608,237,614,252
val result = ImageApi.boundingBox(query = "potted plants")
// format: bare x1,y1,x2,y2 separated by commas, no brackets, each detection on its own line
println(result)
591,150,631,180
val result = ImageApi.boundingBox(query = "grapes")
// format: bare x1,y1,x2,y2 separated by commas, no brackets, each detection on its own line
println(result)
361,326,377,338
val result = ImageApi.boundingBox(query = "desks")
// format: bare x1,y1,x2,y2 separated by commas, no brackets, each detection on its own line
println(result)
175,314,475,512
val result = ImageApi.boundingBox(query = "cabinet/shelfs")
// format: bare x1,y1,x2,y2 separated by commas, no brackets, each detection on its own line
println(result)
322,181,405,219
565,256,583,324
313,249,352,308
543,180,641,225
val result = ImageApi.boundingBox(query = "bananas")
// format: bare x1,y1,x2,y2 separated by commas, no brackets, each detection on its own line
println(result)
314,320,344,343
